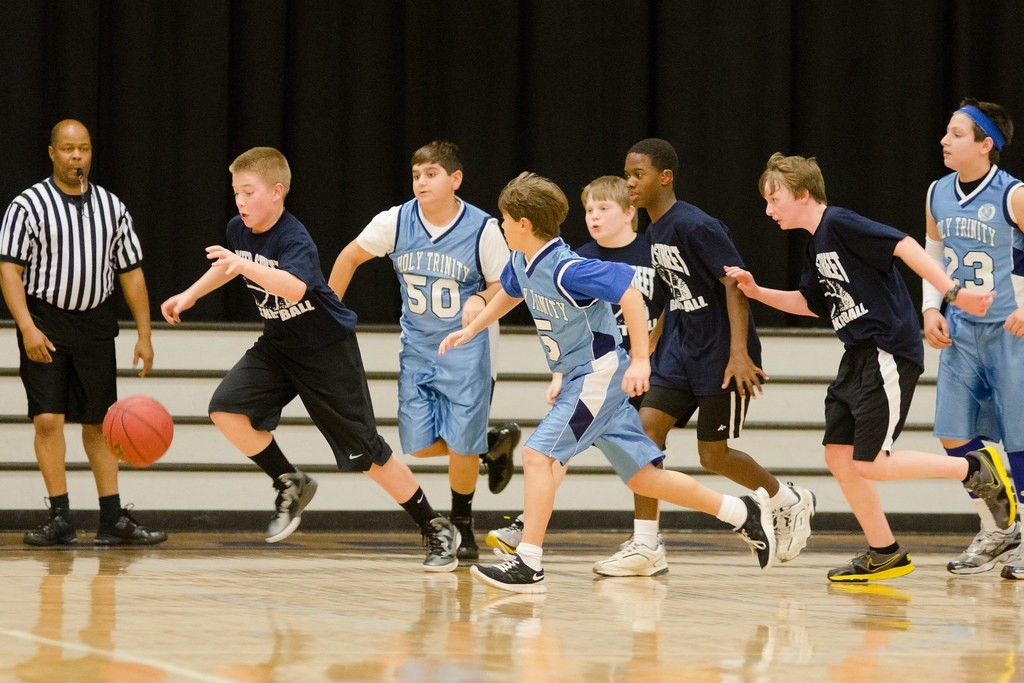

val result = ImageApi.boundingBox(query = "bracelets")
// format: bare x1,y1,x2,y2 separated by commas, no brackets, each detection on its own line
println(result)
471,293,486,306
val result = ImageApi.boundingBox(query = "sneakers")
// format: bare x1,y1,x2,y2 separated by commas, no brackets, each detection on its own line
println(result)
946,521,1024,580
485,513,524,554
415,511,462,571
592,536,669,577
447,516,479,560
23,507,77,546
827,543,914,582
732,487,776,570
263,465,318,543
94,508,169,544
964,444,1017,529
771,482,817,563
470,552,544,594
480,422,521,494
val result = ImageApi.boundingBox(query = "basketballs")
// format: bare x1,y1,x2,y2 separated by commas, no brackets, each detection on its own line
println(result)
101,396,174,467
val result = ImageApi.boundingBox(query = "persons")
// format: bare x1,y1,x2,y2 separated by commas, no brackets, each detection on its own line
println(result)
722,153,1015,583
0,119,168,546
327,140,521,566
922,102,1024,580
438,172,776,595
486,139,818,576
161,146,463,572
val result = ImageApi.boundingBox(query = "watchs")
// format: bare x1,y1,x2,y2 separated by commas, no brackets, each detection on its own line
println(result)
944,284,961,305
